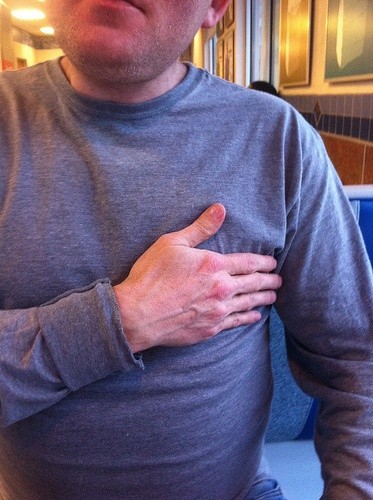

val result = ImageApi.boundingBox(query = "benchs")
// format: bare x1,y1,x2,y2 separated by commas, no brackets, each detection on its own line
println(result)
260,183,373,500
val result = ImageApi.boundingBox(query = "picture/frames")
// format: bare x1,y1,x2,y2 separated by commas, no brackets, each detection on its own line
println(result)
245,0,272,89
277,0,313,89
202,0,234,84
324,0,372,83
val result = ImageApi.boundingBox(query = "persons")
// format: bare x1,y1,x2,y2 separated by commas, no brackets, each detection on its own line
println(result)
0,0,373,499
